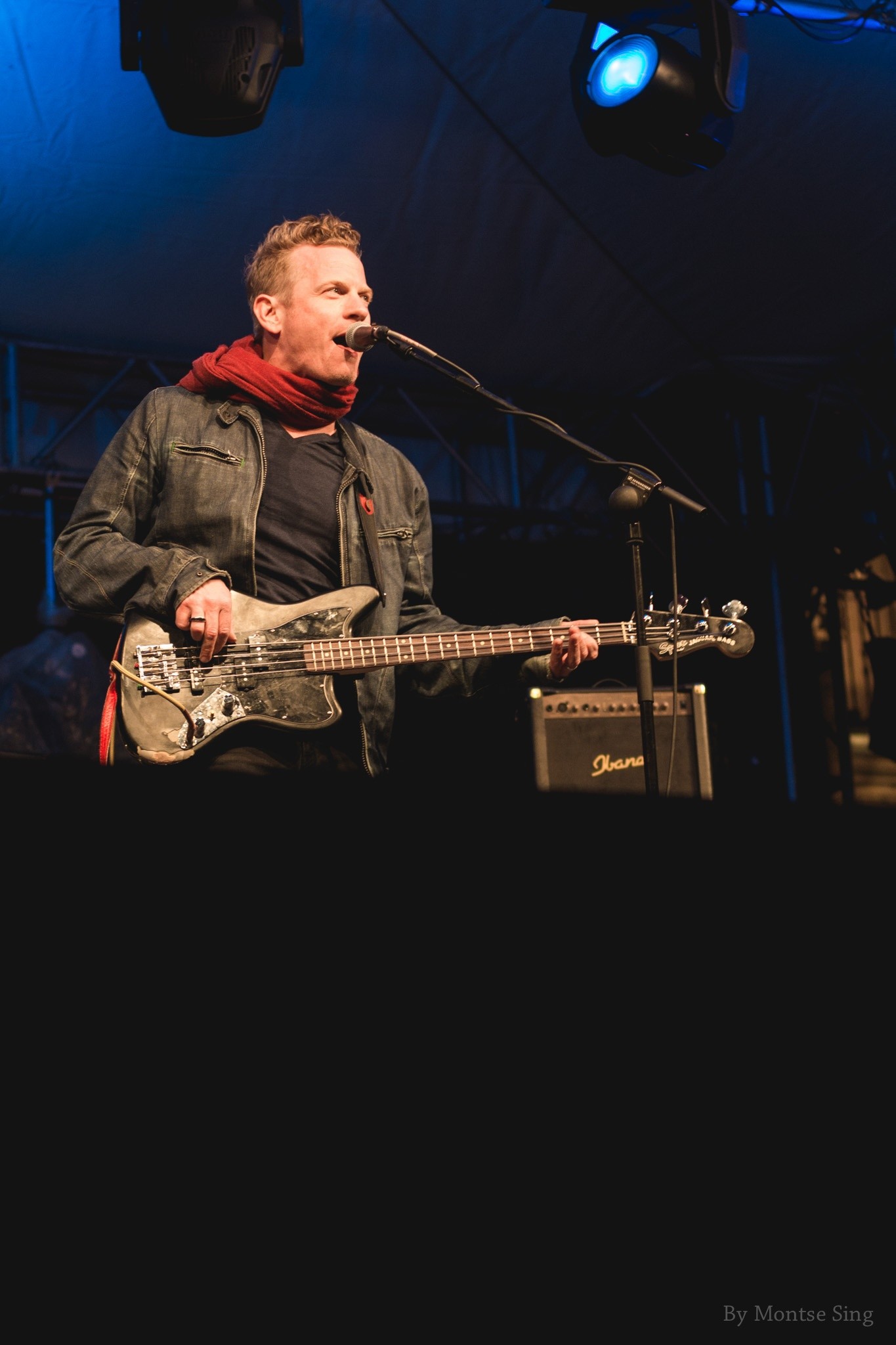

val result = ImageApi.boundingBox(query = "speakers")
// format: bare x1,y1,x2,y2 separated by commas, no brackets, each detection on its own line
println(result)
528,685,714,801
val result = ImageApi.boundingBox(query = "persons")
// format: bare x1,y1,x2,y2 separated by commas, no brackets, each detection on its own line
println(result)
51,212,603,792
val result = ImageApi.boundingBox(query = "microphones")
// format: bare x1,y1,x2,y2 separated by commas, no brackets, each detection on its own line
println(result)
345,321,389,352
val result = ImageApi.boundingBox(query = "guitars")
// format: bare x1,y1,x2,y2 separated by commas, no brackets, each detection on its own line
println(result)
117,584,756,769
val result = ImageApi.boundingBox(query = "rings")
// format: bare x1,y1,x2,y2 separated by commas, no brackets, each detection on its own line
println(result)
564,665,579,672
191,616,207,623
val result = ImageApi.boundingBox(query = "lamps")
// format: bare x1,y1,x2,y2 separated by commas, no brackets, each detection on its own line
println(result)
119,0,304,135
572,21,752,174
